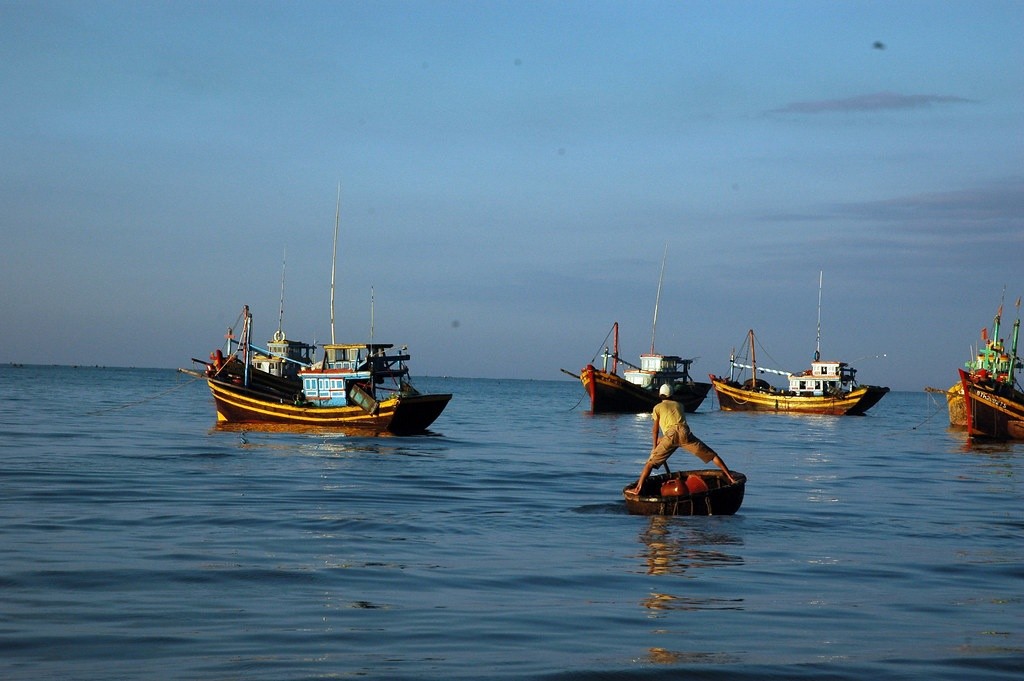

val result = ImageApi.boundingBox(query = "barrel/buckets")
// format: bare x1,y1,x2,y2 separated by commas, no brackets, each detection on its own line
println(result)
660,478,684,496
684,475,709,495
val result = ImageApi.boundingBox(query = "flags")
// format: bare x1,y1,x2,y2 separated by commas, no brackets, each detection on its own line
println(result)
981,328,987,340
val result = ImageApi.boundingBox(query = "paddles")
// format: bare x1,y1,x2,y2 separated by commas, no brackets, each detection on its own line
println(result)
663,460,671,474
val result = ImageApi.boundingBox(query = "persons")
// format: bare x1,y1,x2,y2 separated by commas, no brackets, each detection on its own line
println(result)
626,384,737,496
294,393,306,406
995,377,1010,397
759,387,763,394
716,376,728,384
769,385,776,396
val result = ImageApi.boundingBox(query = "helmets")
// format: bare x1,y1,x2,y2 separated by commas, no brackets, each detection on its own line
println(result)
659,384,674,397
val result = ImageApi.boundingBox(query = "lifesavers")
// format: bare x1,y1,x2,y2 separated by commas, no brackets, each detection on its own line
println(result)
989,341,1004,352
273,330,286,341
813,350,820,361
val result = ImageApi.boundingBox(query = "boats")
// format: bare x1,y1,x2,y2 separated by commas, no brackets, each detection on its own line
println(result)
578,245,712,417
956,285,1024,442
190,182,454,435
706,270,890,418
623,467,748,518
